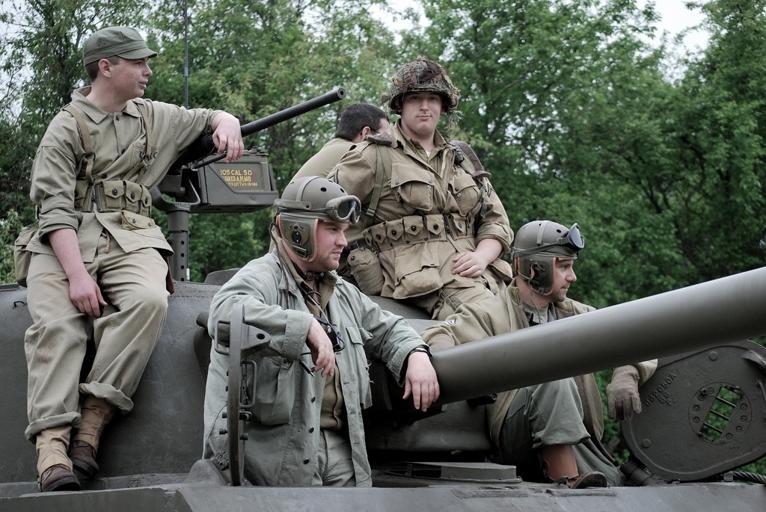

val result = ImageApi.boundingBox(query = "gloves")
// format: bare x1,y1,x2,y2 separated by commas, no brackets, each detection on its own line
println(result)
606,365,642,422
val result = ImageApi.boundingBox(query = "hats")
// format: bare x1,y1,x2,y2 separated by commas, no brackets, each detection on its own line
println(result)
82,26,158,67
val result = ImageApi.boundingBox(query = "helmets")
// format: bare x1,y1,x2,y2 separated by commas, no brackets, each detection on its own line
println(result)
273,175,351,223
387,58,459,111
514,220,578,260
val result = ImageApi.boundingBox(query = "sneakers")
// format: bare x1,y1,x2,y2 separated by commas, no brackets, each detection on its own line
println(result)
37,463,81,491
67,439,100,479
548,470,608,489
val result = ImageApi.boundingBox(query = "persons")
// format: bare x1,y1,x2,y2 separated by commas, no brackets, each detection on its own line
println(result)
420,220,658,490
202,175,441,486
13,26,245,490
325,60,515,322
296,103,392,178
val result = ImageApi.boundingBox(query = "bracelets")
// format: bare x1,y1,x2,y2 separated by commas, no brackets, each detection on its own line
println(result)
409,348,431,356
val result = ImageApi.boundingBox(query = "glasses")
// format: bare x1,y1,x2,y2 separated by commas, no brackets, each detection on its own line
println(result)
327,194,362,225
562,222,585,251
